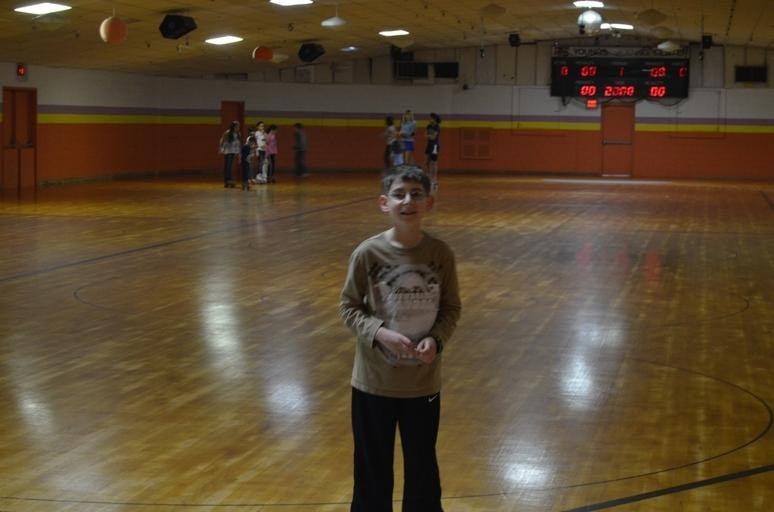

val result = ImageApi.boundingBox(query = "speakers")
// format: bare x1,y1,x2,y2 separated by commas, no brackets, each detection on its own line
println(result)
298,44,325,62
159,15,197,39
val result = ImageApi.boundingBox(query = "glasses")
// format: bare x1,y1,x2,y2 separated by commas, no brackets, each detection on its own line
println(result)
387,191,429,201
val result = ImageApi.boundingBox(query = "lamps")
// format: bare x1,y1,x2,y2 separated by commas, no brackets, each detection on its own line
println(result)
577,7,603,36
100,6,125,45
656,29,683,51
318,2,348,27
270,45,289,63
252,37,273,62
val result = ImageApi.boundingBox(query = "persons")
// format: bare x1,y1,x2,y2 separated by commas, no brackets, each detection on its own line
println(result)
382,109,417,175
218,120,278,191
339,165,462,511
292,122,310,178
422,112,441,191
